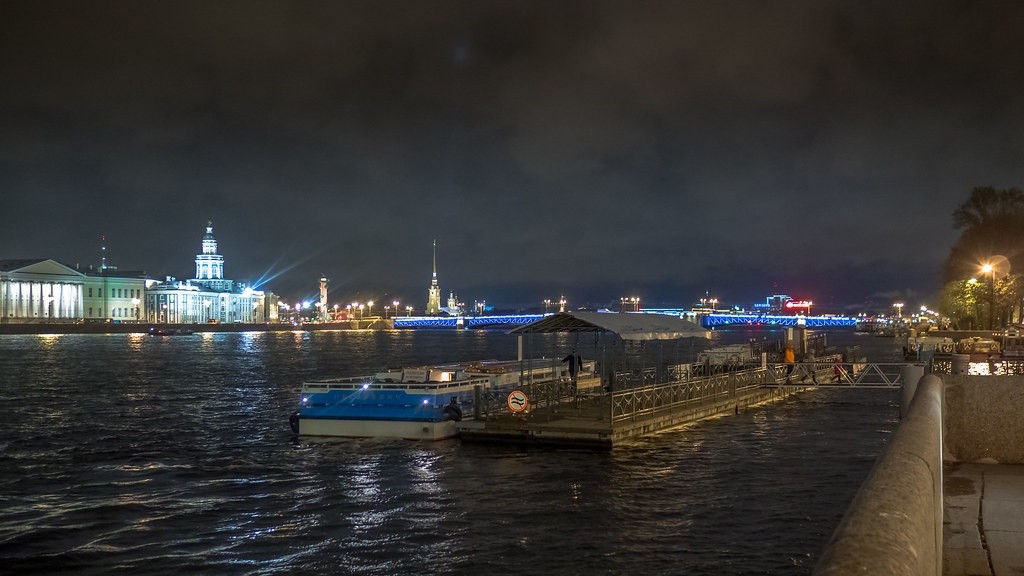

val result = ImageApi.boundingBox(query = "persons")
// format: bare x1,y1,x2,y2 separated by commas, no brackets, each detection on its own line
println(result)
562,349,583,396
785,341,795,384
831,355,843,383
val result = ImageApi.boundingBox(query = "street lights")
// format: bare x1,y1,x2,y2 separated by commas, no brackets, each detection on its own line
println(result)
347,305,351,320
394,302,399,318
359,305,363,320
352,303,357,317
368,302,371,317
982,266,994,330
384,306,390,318
334,305,337,319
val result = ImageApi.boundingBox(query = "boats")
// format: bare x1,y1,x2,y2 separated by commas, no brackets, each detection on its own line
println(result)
149,328,195,335
290,356,601,442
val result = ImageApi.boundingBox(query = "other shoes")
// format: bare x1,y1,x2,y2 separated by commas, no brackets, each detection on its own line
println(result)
830,379,832,382
813,381,818,384
786,381,792,384
838,380,843,382
800,379,804,383
